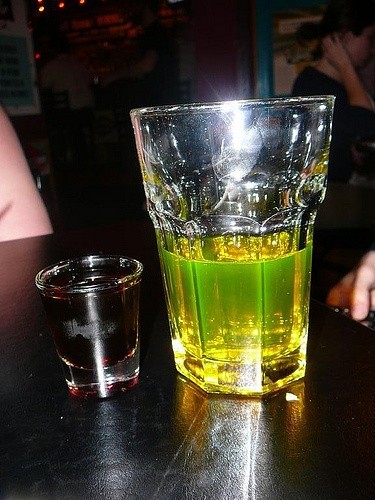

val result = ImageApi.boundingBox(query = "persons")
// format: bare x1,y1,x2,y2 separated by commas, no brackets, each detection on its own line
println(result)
37,0,180,147
326,239,375,330
0,106,54,243
289,0,375,183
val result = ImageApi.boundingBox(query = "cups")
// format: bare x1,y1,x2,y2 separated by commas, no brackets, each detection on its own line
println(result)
129,95,336,396
34,256,143,399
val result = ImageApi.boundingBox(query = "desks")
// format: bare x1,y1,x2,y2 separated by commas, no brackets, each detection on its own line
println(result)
0,255,375,500
